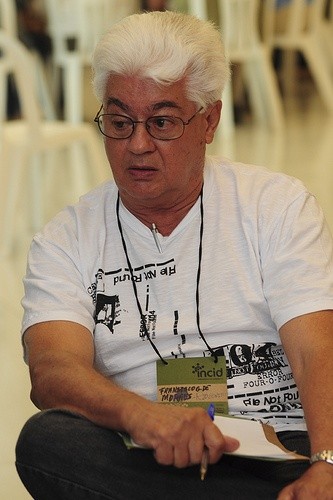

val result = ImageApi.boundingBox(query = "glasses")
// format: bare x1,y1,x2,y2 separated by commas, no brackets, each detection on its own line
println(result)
93,102,204,141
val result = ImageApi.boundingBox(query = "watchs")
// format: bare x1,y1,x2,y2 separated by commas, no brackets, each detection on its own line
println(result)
309,450,333,464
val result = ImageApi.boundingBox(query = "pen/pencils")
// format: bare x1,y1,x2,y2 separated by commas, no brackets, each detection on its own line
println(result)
201,404,215,480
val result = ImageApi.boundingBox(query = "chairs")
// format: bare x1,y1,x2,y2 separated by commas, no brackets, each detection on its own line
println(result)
0,0,333,142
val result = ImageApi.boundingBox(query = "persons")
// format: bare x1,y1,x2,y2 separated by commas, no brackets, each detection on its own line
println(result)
8,0,54,121
15,12,333,500
